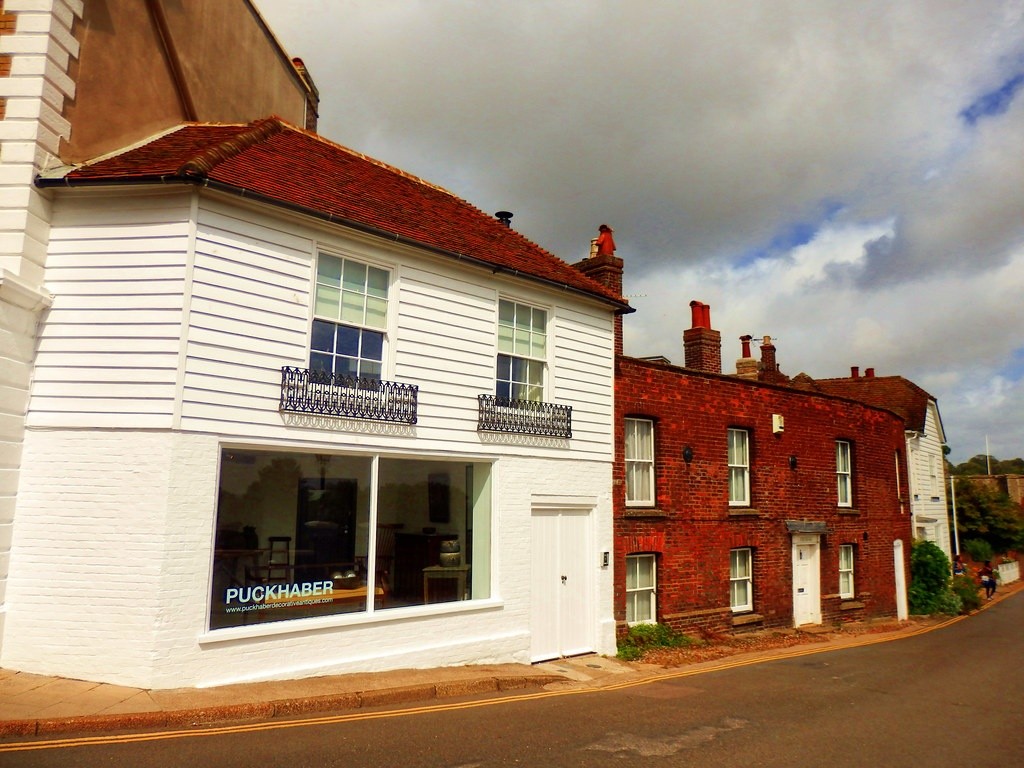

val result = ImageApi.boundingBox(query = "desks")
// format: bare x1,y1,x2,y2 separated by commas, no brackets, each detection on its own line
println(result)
422,564,471,604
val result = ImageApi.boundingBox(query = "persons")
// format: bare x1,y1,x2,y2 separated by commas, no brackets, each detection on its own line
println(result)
952,555,965,576
978,559,997,601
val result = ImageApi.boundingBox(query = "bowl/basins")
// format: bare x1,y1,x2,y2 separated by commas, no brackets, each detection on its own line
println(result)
331,570,357,578
422,527,436,535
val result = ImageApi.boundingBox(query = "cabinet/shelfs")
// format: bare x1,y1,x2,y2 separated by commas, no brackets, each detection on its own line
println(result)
393,532,459,599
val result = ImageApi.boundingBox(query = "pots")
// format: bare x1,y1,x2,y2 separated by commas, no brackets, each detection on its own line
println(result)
440,540,460,553
439,553,461,567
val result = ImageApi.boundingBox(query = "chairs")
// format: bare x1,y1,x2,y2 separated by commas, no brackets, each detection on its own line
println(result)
267,536,292,588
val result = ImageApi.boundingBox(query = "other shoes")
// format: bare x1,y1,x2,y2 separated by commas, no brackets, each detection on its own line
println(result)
988,598,992,600
990,596,993,600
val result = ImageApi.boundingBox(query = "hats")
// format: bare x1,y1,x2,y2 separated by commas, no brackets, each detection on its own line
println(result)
953,555,960,561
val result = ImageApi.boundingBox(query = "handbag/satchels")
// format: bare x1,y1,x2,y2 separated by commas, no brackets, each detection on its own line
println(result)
981,576,989,581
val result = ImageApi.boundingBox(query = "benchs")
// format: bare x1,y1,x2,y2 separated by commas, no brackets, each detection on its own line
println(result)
244,555,394,626
212,549,314,624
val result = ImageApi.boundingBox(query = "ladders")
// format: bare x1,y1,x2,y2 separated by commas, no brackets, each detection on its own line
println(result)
268,537,292,587
